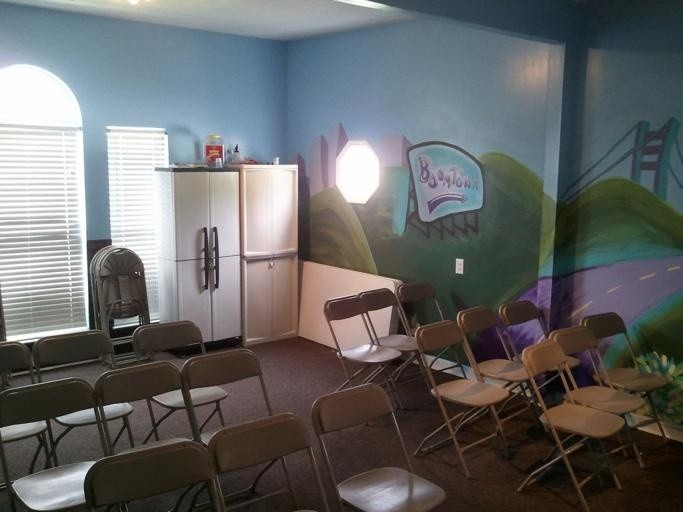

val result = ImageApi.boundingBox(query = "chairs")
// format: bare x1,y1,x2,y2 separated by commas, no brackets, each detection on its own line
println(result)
0,321,329,511
311,281,671,512
89,246,149,367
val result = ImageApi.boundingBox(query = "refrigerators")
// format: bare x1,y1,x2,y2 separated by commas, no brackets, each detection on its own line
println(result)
149,168,240,346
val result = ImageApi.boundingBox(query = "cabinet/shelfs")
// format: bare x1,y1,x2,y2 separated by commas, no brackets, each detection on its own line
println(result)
227,162,301,347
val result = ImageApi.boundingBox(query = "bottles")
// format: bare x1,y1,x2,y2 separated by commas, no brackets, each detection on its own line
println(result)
226,143,241,165
200,133,226,166
215,158,222,168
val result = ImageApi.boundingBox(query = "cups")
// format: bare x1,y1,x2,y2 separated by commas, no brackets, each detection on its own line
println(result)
272,157,281,164
